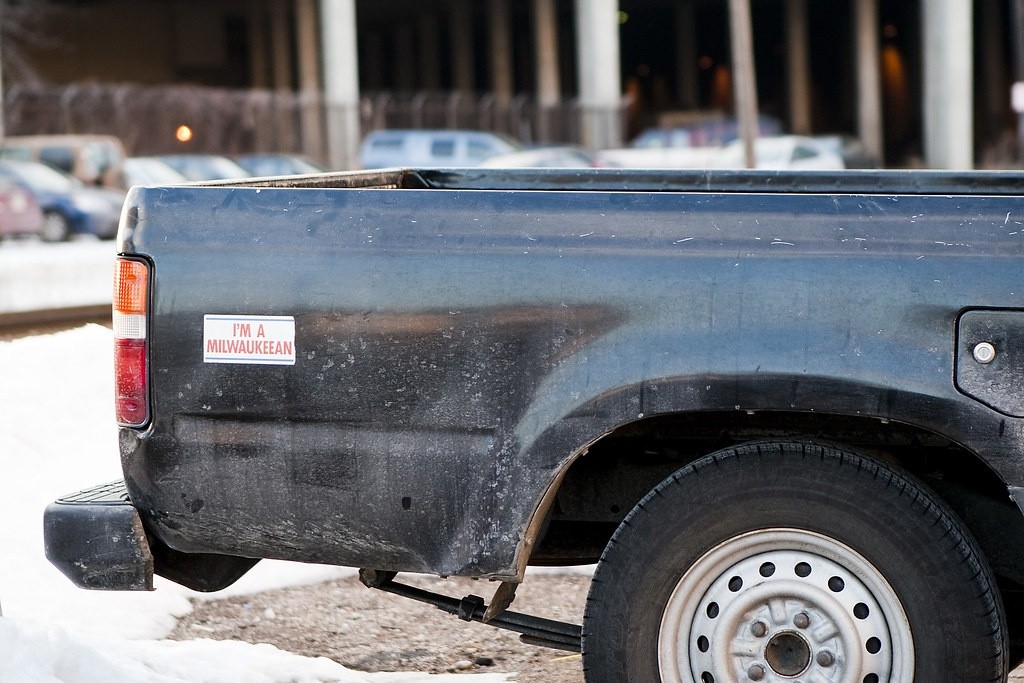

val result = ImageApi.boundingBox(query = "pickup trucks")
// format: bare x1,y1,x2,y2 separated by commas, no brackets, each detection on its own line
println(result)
41,162,1024,683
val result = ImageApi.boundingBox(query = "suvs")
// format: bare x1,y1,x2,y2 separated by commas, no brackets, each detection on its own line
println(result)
355,128,528,169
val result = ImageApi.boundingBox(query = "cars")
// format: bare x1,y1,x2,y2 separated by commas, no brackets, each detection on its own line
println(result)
479,115,887,170
1,135,330,244
